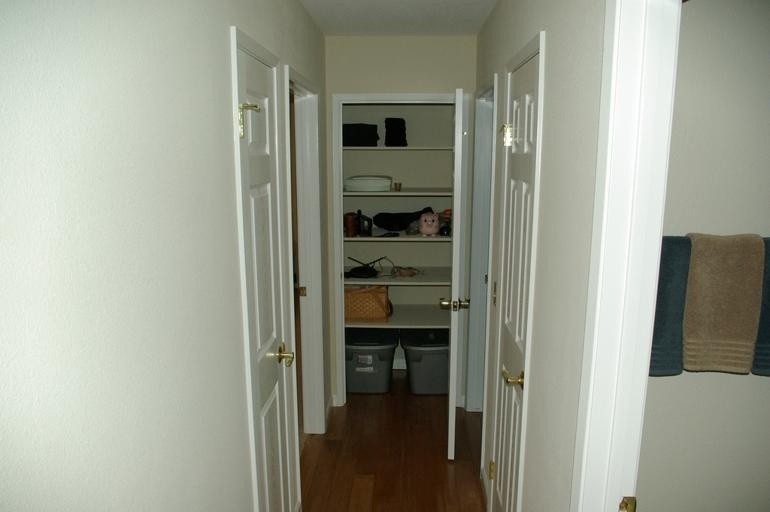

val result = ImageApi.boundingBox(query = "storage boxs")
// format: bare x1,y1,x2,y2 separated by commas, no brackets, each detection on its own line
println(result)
343,123,378,146
399,329,449,394
345,329,399,393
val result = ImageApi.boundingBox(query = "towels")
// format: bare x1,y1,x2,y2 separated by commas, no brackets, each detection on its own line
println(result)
681,233,765,374
751,236,770,376
647,236,691,376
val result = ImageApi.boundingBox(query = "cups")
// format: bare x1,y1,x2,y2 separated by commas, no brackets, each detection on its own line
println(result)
344,212,359,238
394,182,402,191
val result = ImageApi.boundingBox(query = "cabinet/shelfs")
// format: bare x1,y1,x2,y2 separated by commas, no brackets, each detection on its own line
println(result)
342,145,454,329
